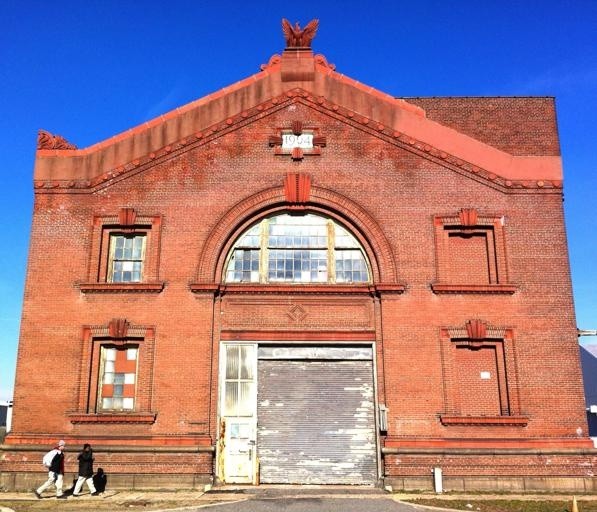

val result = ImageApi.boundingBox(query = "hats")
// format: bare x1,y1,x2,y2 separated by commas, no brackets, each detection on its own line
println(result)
84,444,90,449
59,440,64,446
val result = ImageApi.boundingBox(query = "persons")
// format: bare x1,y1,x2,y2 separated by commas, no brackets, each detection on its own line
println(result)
72,444,99,497
35,440,68,499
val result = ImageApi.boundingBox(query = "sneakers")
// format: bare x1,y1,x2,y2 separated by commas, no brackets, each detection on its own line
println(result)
57,495,67,499
73,492,100,496
34,490,41,500
